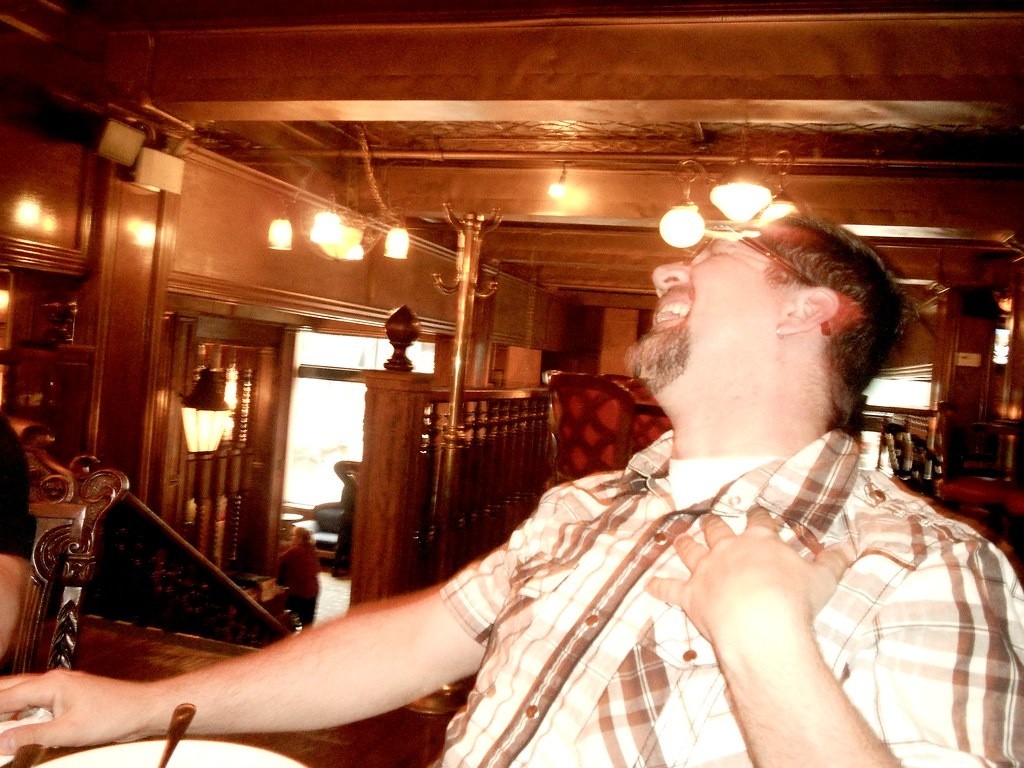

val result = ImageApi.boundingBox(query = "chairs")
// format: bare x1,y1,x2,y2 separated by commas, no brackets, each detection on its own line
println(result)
538,372,675,492
0,413,132,675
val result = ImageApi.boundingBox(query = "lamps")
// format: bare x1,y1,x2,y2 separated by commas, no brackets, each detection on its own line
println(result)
657,133,804,250
265,165,411,262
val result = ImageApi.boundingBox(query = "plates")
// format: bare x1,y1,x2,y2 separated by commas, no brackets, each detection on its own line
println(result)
25,738,309,768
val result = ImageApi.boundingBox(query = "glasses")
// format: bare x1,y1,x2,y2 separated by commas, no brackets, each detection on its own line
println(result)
692,224,831,336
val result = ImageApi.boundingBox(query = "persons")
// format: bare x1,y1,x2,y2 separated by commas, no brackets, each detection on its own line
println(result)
1,210,1024,768
277,526,321,632
0,414,31,669
